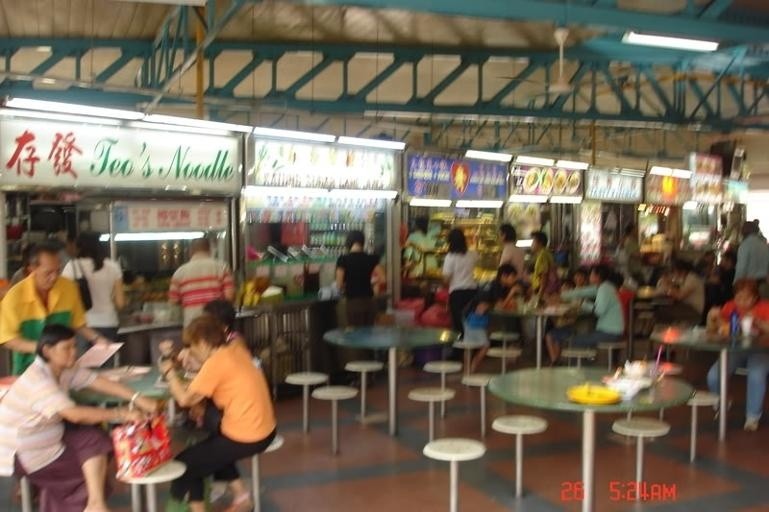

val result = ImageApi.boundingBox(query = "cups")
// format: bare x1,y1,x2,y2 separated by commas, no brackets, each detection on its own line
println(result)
740,316,753,336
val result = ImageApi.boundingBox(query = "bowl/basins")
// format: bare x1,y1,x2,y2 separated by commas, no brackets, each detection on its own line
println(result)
607,379,643,400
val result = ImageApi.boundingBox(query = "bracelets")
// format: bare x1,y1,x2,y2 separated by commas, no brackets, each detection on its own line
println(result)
129,389,141,404
161,366,174,381
89,331,103,344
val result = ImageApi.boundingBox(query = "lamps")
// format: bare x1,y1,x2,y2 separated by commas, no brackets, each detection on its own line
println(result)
620,27,721,53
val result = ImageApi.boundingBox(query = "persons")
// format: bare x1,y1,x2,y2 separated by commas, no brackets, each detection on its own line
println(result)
59,232,125,369
2,324,162,511
165,236,239,340
158,316,276,512
121,272,172,324
401,217,769,378
335,227,387,393
704,273,769,430
2,236,114,375
158,299,239,508
241,265,284,309
8,232,79,288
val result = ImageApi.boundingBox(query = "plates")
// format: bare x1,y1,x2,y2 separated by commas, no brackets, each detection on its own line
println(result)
539,167,554,194
567,169,581,194
554,168,568,194
605,376,652,390
566,385,619,404
522,167,541,194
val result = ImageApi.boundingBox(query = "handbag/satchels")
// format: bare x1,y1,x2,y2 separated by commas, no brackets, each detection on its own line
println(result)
540,271,560,295
72,258,92,310
112,414,172,481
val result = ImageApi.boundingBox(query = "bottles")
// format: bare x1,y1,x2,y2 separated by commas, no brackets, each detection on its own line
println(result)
731,310,738,337
310,223,364,231
310,231,347,246
311,246,347,258
245,196,379,223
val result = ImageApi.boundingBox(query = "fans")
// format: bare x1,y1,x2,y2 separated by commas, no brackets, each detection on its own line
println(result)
496,26,625,96
